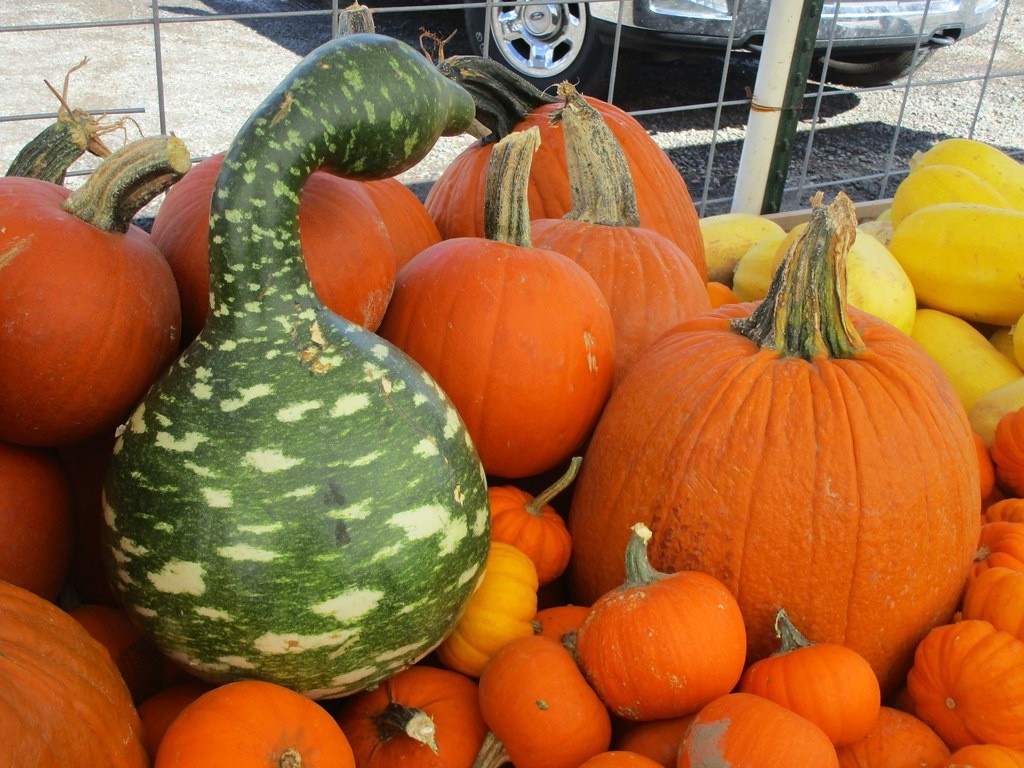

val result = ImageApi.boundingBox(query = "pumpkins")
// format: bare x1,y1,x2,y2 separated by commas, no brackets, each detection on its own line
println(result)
0,37,1024,767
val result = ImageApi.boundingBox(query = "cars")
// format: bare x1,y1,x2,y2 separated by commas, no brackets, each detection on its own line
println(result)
464,0,999,88
